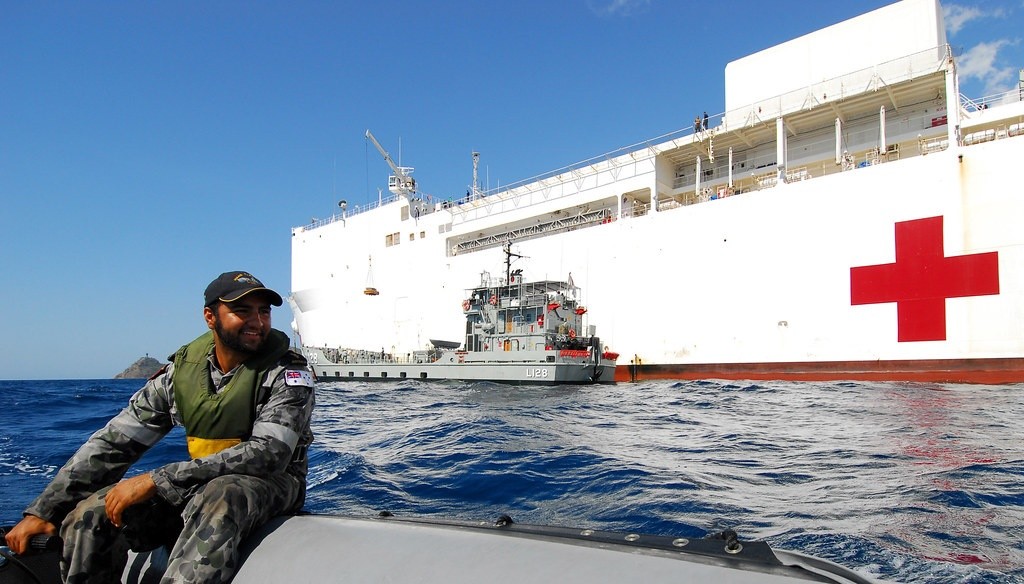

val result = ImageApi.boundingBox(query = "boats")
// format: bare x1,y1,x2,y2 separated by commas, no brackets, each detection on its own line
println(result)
304,238,621,389
288,0,1024,383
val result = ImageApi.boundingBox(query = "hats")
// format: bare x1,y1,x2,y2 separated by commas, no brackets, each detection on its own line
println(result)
204,271,282,307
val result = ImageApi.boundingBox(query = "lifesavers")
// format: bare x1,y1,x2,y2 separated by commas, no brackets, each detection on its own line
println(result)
490,296,496,305
511,277,514,282
569,329,575,338
462,300,470,311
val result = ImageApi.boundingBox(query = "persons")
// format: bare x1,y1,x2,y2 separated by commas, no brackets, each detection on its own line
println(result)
635,354,639,365
448,195,452,208
414,206,420,218
603,216,612,224
5,271,316,584
711,192,717,201
703,112,708,131
382,347,384,359
694,116,701,133
978,103,988,110
443,200,447,208
466,190,470,202
545,334,594,350
